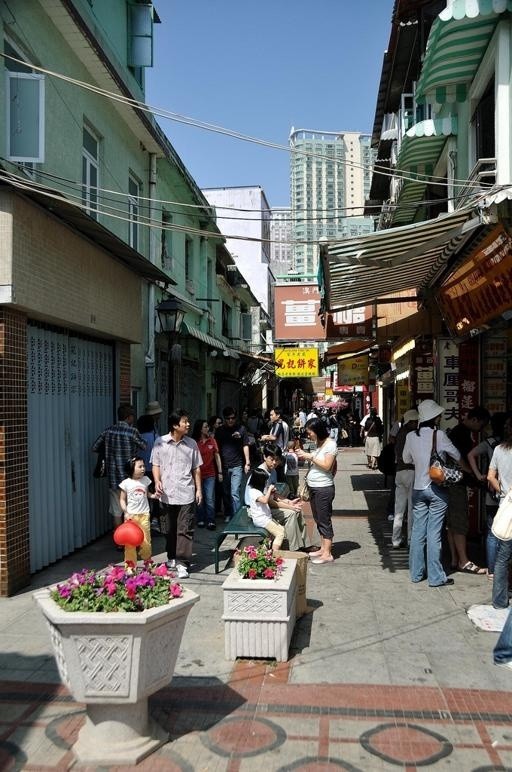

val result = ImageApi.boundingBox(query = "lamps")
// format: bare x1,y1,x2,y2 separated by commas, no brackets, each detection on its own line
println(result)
154,293,190,352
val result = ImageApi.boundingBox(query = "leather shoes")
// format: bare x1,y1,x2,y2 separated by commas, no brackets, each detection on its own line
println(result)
309,551,333,564
444,579,454,585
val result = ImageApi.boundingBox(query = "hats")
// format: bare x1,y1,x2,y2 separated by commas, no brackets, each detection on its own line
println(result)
145,401,163,415
417,399,445,429
403,410,419,425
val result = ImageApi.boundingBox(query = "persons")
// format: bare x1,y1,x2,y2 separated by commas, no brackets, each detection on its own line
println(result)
294,407,357,448
466,486,512,669
391,399,512,586
360,406,384,469
92,400,339,578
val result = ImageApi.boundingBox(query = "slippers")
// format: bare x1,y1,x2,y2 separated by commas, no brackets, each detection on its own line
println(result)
458,561,485,573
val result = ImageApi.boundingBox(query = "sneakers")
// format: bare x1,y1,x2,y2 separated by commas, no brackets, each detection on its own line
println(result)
176,564,188,578
198,516,230,530
167,559,175,568
150,523,160,533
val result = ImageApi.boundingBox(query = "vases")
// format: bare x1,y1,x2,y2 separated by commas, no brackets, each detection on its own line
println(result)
221,560,300,665
30,571,200,766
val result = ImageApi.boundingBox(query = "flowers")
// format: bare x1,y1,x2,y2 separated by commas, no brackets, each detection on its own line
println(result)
44,555,186,614
233,536,288,581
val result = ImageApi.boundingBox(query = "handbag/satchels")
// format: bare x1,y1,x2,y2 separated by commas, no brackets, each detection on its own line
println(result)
297,480,310,501
93,442,106,478
430,430,464,489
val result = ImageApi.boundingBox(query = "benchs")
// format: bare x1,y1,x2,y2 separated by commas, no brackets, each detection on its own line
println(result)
214,504,273,574
